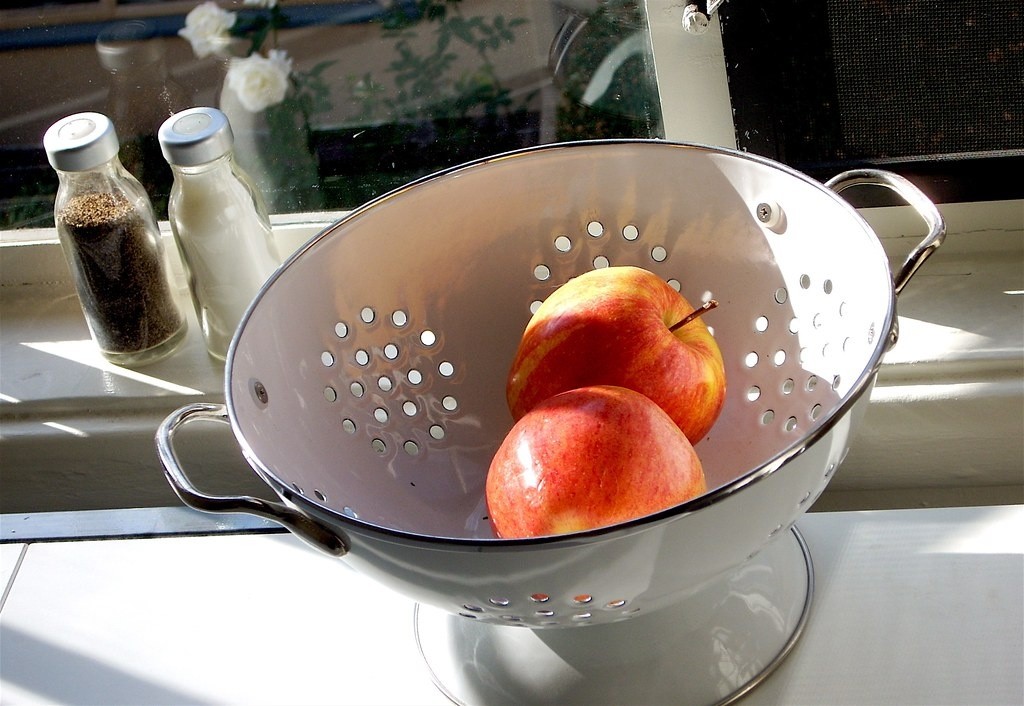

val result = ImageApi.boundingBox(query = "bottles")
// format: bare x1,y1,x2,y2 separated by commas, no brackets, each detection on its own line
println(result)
95,18,195,214
42,111,190,368
158,106,283,361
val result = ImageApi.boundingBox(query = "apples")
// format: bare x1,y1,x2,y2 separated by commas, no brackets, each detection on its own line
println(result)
483,384,709,603
505,266,727,448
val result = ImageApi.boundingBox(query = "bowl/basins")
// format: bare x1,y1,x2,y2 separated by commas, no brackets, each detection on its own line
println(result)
153,137,948,630
